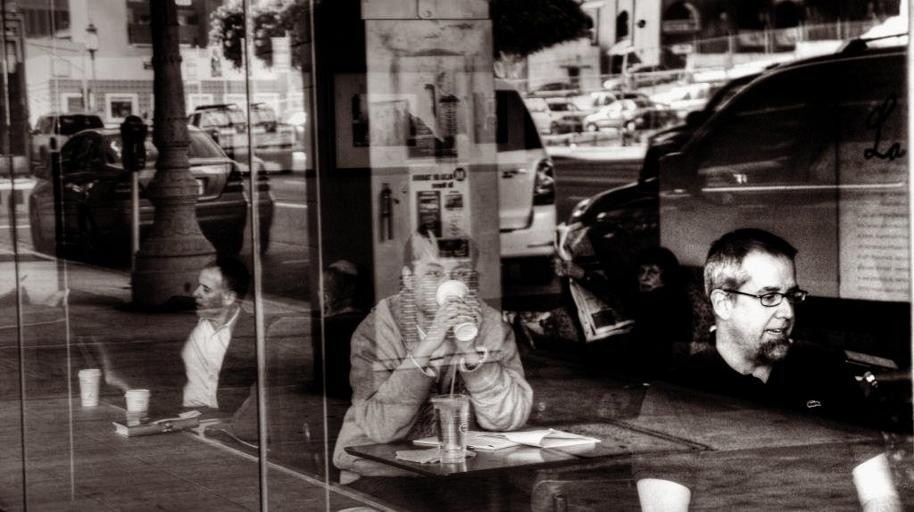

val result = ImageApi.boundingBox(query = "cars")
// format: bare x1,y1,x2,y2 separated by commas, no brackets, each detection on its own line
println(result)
604,62,678,92
581,98,677,133
26,115,249,256
528,80,581,100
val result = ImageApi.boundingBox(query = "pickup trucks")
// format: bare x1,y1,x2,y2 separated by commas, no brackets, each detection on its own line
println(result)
186,102,295,171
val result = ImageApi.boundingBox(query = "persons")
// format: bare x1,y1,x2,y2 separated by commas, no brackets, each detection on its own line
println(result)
167,256,256,441
332,222,533,512
629,228,903,512
552,246,695,392
319,260,358,315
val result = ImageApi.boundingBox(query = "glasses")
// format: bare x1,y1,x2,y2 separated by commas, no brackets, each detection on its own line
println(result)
722,285,808,307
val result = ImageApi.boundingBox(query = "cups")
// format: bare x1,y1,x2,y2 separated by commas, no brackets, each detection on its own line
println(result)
124,387,152,413
429,391,472,467
78,365,104,408
436,278,480,341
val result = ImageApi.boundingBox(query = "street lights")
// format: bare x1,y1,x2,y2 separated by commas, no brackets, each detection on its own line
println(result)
79,23,101,120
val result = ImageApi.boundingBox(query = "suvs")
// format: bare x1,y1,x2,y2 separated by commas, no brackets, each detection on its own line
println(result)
29,111,108,165
523,98,590,135
493,75,561,274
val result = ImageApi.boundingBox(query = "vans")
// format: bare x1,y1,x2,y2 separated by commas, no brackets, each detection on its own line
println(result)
554,33,908,384
